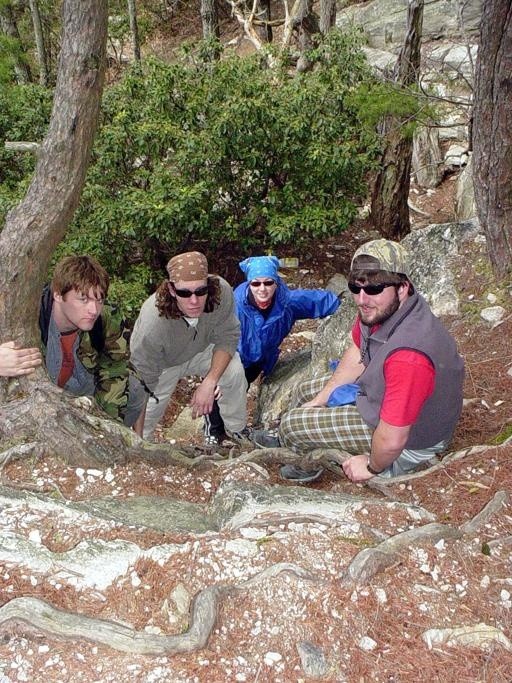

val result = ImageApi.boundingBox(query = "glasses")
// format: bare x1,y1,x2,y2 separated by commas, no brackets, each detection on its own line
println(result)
348,282,397,295
169,282,209,297
249,281,276,286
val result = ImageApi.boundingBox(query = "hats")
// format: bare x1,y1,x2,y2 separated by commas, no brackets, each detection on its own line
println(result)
350,238,410,274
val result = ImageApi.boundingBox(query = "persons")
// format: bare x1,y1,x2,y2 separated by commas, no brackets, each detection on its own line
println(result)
1,251,130,427
199,252,342,445
116,249,255,448
249,237,466,485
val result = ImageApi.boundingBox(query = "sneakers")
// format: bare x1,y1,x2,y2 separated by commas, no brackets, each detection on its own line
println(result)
254,429,280,450
279,464,324,482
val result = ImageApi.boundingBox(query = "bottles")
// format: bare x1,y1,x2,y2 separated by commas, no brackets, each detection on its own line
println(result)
274,256,300,269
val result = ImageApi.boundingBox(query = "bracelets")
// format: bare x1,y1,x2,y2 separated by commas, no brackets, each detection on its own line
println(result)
366,457,386,475
